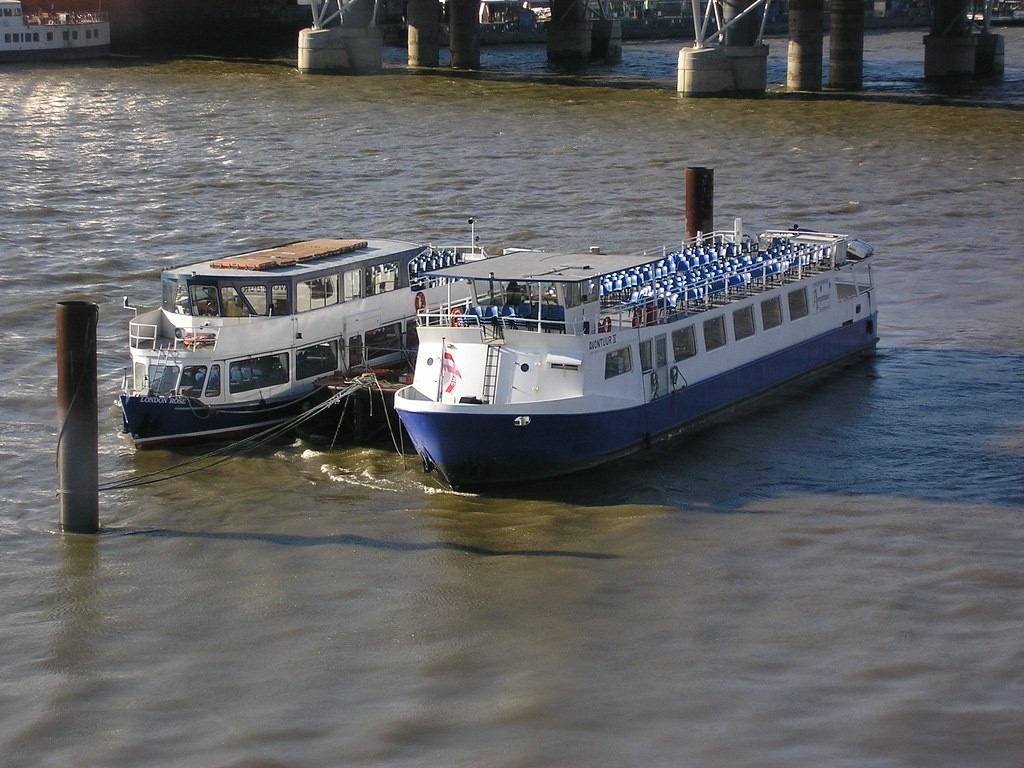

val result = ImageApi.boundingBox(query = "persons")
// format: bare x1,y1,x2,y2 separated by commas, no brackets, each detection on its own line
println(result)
506,281,521,306
204,287,217,308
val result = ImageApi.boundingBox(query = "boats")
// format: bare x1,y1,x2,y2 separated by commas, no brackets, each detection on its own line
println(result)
0,0,112,68
119,216,498,452
967,0,1024,23
394,217,881,493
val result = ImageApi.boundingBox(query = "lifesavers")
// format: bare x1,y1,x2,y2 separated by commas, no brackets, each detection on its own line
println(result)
450,308,462,327
184,335,214,347
602,317,612,333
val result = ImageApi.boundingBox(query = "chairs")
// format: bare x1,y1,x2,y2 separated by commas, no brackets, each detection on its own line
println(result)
454,303,566,341
169,365,264,392
371,250,461,290
574,242,831,325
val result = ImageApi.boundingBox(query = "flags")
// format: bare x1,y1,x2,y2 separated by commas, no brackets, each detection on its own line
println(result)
443,349,462,397
